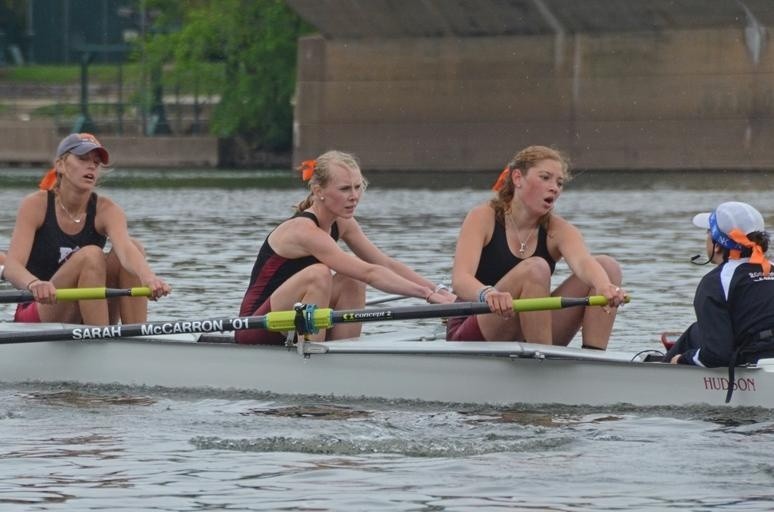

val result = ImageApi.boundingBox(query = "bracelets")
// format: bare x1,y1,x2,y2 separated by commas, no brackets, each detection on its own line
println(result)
476,284,497,303
25,278,41,292
433,282,449,295
426,290,436,302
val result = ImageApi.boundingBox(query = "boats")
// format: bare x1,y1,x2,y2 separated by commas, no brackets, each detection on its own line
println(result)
0,321,774,414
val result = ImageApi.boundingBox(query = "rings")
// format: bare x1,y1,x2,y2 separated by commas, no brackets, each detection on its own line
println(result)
614,285,621,293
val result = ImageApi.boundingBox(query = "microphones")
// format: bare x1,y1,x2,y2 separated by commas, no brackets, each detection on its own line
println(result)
691,235,716,265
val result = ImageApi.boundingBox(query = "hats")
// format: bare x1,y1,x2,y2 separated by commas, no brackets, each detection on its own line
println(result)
57,133,109,164
692,201,765,250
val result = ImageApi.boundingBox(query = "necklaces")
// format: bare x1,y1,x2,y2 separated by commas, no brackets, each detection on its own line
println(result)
56,189,88,224
507,211,538,255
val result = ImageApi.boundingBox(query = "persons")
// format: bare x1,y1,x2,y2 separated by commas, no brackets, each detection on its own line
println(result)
1,130,174,327
232,148,459,346
444,143,626,352
645,199,774,370
